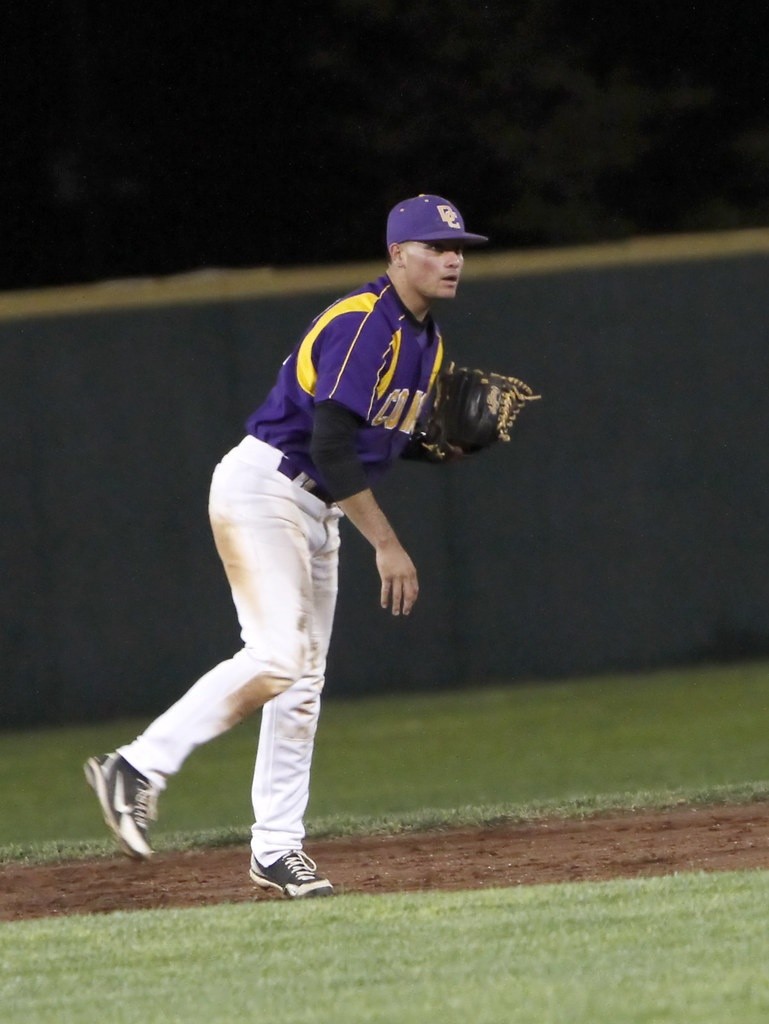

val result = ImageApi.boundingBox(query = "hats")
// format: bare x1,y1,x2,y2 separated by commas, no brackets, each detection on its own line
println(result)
386,193,488,249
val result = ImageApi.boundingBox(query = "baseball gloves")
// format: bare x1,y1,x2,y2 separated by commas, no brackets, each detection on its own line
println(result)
416,362,542,463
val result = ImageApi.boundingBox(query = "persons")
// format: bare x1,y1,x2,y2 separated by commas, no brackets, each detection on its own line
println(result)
85,195,517,899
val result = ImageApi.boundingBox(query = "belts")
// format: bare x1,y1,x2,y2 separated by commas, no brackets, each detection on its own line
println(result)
277,456,336,506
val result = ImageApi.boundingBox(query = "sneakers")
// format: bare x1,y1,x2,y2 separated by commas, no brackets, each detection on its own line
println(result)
248,850,334,898
83,752,161,863
